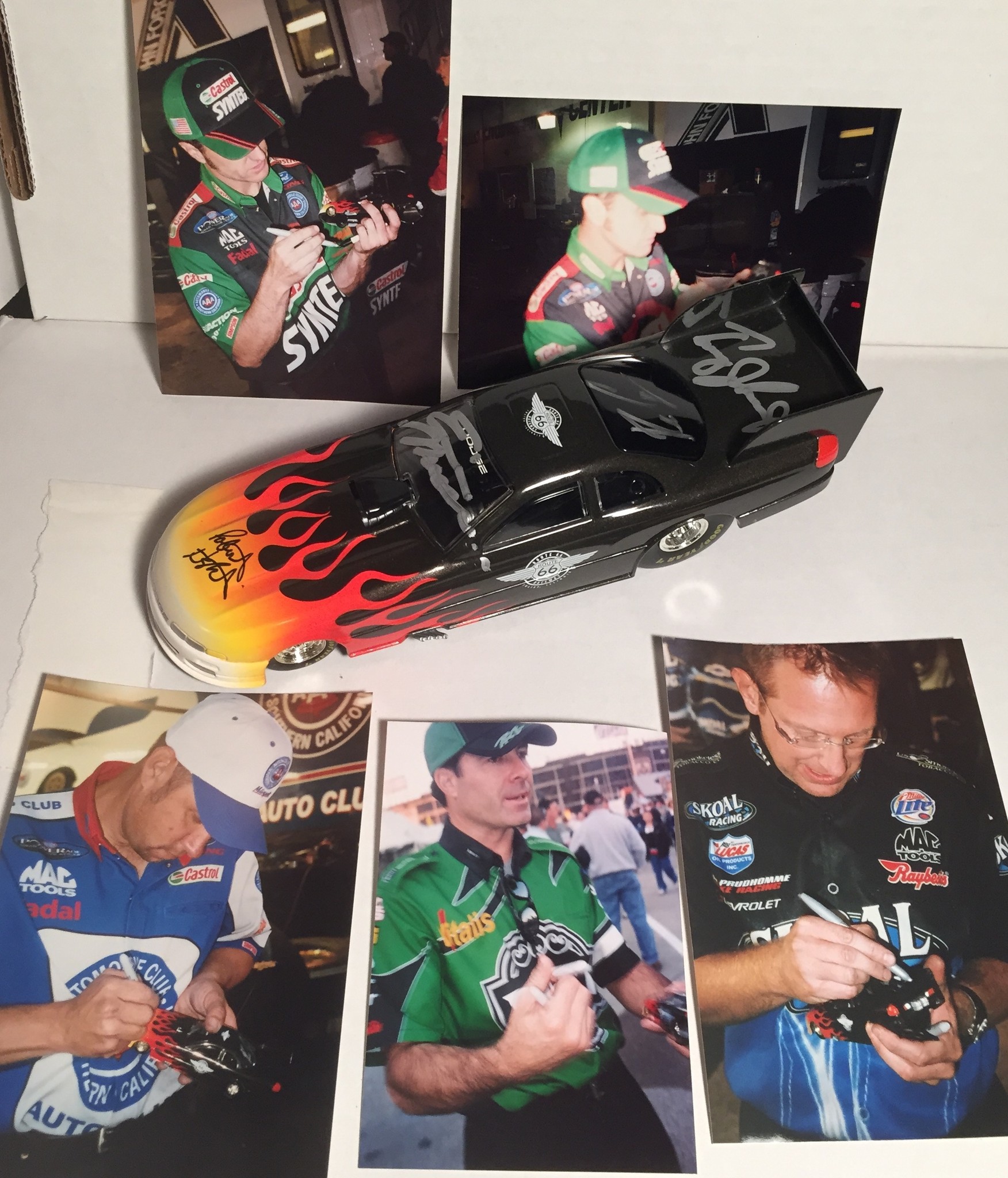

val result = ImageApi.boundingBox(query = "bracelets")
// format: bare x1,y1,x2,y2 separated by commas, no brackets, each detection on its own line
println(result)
952,984,989,1044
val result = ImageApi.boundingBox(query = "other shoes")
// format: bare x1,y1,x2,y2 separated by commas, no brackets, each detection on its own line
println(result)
652,962,663,972
659,884,666,895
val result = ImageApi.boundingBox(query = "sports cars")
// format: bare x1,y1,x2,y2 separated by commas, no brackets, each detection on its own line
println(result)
144,267,884,691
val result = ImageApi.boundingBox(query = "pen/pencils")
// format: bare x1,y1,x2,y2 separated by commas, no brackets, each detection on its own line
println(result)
266,225,337,248
120,953,142,984
799,892,913,984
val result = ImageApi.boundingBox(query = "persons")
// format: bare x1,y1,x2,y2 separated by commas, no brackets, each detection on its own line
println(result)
523,128,714,373
1,643,1007,1178
159,32,446,403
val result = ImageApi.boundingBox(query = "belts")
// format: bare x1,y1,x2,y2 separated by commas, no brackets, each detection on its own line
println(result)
10,1117,143,1155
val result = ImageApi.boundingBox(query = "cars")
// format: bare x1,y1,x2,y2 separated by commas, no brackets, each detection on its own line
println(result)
319,167,428,231
808,969,945,1045
127,1006,283,1101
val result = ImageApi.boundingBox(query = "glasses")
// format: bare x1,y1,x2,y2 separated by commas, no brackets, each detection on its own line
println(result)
745,677,886,750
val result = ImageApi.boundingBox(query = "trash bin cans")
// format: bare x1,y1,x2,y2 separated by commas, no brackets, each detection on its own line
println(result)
322,128,409,201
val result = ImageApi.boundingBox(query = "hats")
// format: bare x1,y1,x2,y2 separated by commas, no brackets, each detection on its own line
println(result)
166,690,296,854
379,32,406,51
162,57,286,160
568,126,698,217
428,722,556,777
584,790,604,806
